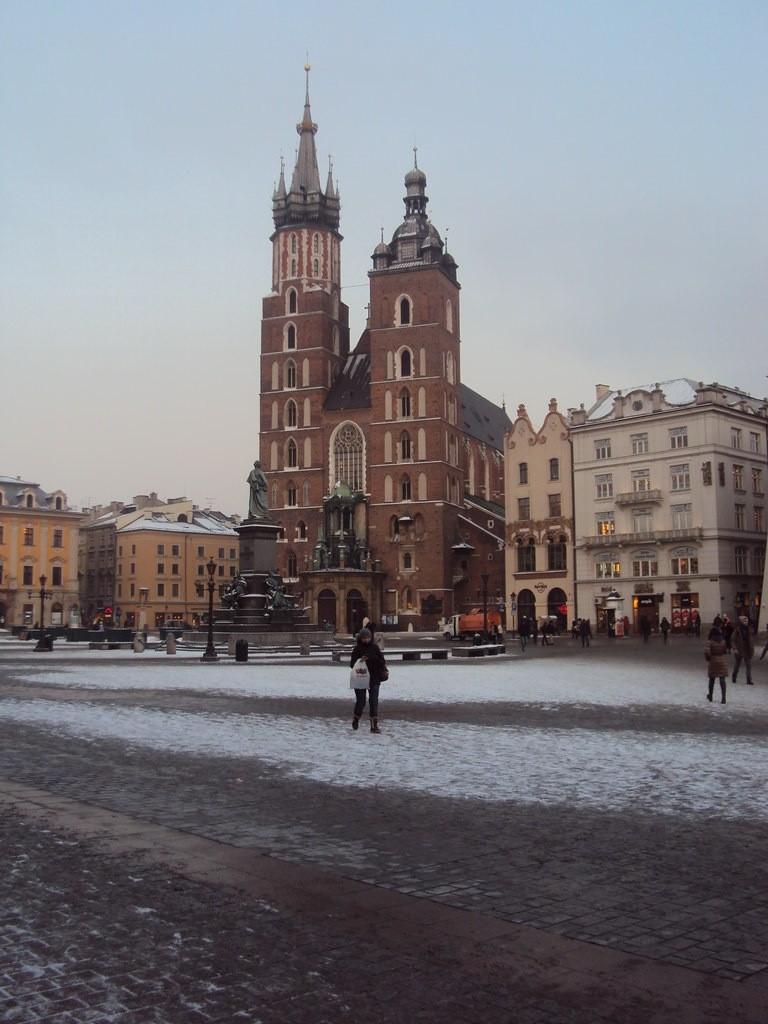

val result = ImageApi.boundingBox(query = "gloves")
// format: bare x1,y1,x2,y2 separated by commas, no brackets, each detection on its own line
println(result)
733,649,739,655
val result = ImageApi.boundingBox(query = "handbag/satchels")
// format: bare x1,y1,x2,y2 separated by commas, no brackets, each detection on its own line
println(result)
350,657,371,690
380,663,389,681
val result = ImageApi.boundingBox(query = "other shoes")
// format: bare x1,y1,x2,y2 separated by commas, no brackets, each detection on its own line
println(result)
747,680,753,685
707,694,712,702
721,700,726,704
732,672,737,683
760,654,765,660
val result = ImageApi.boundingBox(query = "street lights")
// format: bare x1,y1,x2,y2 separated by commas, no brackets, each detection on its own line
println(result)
27,573,54,648
510,591,517,639
475,566,501,635
194,555,230,662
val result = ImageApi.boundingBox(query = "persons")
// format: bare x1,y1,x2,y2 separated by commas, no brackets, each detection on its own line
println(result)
608,615,630,639
660,617,669,644
704,626,729,704
520,617,555,651
265,569,288,610
730,616,754,685
225,570,248,610
713,613,730,636
246,460,272,520
695,614,701,636
571,617,592,647
491,621,503,645
640,615,651,641
351,629,389,732
760,623,768,660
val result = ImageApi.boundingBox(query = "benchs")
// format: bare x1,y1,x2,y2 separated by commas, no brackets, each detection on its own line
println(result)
12,625,505,661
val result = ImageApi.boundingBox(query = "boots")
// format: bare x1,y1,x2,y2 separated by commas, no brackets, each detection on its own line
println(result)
368,717,380,733
352,715,360,730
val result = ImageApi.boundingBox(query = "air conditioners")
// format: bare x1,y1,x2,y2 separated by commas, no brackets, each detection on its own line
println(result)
594,598,603,604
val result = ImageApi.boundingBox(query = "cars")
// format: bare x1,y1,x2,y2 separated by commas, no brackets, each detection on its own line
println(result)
159,619,193,640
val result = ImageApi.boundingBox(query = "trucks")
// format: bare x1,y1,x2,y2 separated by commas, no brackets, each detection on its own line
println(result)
442,607,502,641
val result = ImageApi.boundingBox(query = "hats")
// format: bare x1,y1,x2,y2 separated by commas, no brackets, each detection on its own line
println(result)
522,615,527,619
738,616,748,623
358,628,371,639
